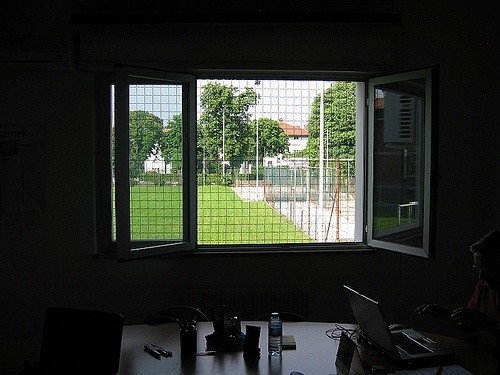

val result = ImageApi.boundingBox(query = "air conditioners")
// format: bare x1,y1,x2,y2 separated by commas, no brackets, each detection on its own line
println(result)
0,32,74,72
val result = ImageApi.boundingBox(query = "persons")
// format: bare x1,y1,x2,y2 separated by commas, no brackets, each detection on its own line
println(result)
415,227,500,358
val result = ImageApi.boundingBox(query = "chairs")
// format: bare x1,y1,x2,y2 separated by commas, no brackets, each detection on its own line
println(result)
155,306,210,323
20,307,124,375
258,312,311,322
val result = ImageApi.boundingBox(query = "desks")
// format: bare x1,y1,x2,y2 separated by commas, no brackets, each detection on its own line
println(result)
115,322,473,375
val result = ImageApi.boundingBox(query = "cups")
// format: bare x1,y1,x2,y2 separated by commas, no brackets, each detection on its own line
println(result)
180,333,197,355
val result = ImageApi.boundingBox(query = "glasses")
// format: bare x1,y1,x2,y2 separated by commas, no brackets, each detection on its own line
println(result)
471,264,496,274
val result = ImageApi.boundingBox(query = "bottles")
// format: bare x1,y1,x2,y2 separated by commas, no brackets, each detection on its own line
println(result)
268,312,282,356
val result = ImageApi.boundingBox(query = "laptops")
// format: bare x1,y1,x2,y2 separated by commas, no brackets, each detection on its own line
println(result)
344,285,456,367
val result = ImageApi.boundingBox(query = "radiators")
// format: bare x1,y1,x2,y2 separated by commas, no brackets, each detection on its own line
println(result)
168,287,312,322
